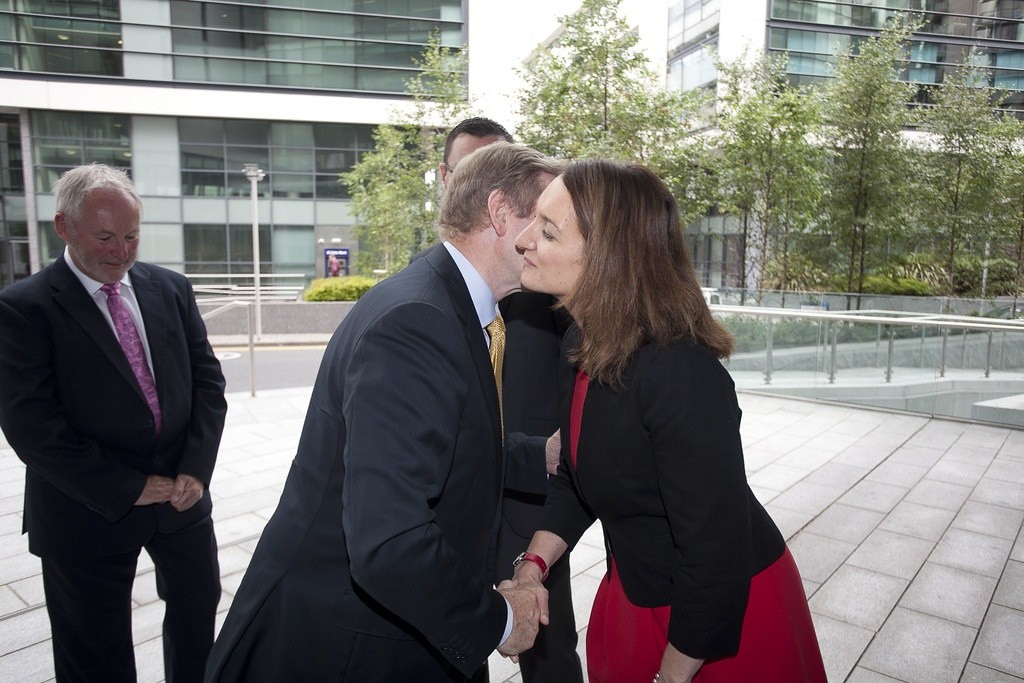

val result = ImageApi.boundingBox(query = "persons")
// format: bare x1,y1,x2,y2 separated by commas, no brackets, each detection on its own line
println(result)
441,117,583,683
199,143,558,683
512,159,827,683
0,163,227,683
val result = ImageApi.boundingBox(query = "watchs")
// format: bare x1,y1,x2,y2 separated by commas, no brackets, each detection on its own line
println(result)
513,551,549,582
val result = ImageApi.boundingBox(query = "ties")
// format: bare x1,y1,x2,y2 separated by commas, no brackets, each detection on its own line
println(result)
99,282,162,440
486,313,506,448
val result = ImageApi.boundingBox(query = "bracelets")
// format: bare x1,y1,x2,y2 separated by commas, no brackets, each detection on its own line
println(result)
653,671,660,683
